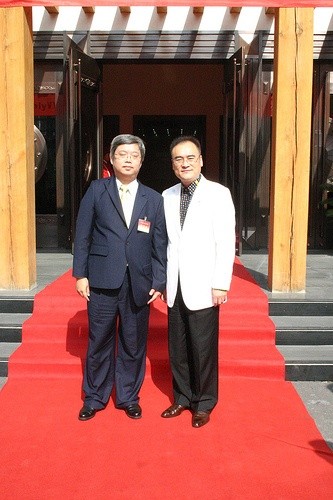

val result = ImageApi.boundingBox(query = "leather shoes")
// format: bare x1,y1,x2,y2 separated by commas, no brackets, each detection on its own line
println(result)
192,409,211,428
78,405,96,421
161,402,187,418
124,403,142,419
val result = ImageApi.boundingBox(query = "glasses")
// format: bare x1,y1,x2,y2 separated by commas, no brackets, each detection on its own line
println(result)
114,151,139,159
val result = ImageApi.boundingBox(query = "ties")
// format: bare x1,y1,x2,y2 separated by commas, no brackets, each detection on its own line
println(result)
120,184,127,204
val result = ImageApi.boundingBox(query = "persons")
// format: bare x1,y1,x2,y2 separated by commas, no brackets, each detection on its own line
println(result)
72,134,168,420
160,136,236,427
